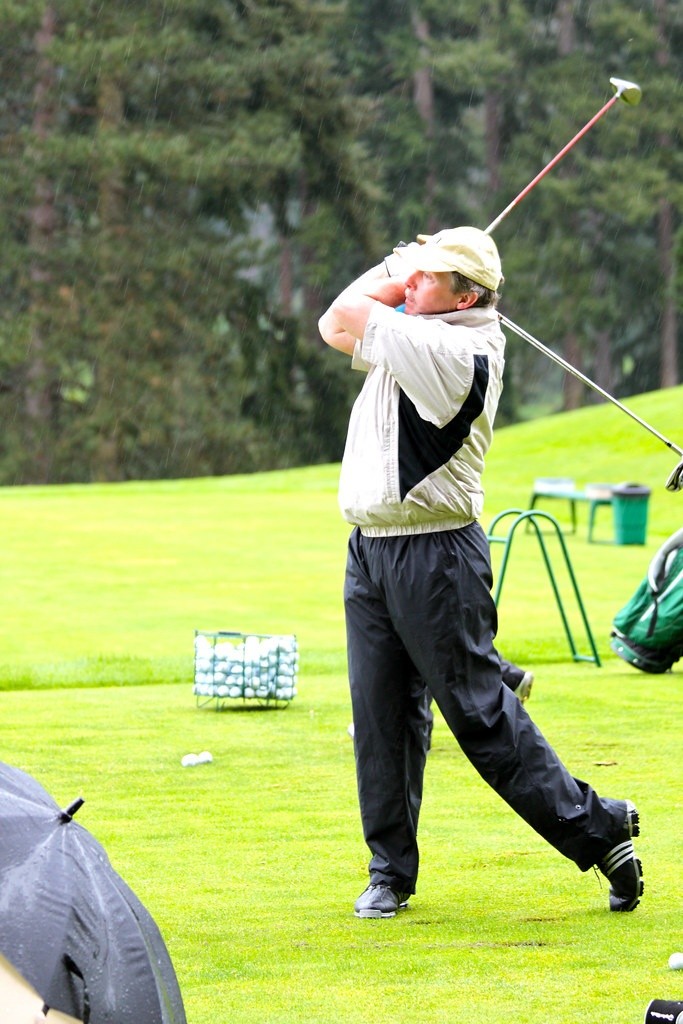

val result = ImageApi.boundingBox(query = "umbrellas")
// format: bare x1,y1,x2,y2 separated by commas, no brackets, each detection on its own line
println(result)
0,756,188,1024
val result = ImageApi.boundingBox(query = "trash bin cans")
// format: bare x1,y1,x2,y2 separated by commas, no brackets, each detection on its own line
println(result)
611,480,651,547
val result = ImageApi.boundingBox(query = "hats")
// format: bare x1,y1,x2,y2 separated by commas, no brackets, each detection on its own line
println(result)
393,226,502,290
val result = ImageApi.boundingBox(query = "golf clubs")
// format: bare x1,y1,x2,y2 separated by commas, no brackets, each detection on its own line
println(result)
496,311,683,492
484,77,642,235
182,752,212,766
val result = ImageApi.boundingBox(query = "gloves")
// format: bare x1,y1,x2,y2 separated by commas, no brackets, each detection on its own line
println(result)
384,241,420,276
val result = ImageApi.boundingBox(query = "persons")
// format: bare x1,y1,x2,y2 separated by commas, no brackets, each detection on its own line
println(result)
319,226,645,921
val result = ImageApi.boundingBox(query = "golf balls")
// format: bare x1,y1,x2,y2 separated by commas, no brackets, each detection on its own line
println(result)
195,631,299,699
668,952,683,970
348,724,354,735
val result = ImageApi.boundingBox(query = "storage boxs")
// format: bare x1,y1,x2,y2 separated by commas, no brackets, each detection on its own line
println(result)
194,628,298,713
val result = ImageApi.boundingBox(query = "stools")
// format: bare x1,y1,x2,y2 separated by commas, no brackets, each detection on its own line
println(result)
525,477,580,535
584,482,614,543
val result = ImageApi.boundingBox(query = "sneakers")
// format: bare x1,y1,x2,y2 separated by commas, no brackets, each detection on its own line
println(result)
501,663,533,701
353,882,411,919
595,799,644,911
